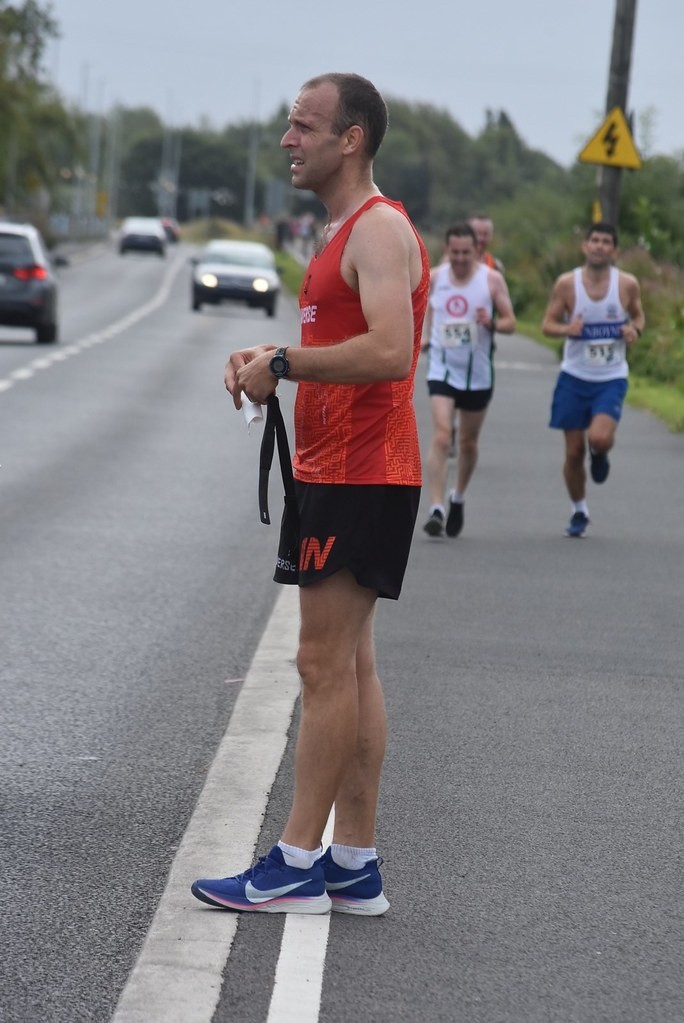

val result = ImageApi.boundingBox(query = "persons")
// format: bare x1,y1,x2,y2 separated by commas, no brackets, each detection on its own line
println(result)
543,224,645,536
439,216,505,459
190,74,431,917
421,222,515,537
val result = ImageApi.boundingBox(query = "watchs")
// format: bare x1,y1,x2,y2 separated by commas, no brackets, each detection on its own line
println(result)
270,346,290,378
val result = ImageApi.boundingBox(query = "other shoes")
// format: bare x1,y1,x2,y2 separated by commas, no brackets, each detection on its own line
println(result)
589,446,609,484
566,512,590,538
445,493,463,538
424,509,443,536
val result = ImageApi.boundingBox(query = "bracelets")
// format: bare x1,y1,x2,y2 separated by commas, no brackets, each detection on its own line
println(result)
491,319,496,330
635,328,641,337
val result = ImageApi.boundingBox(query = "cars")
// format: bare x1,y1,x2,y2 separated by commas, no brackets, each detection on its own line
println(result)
159,218,180,243
188,240,284,317
119,216,166,256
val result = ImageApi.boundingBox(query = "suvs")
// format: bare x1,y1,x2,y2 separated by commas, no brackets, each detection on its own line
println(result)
0,223,60,344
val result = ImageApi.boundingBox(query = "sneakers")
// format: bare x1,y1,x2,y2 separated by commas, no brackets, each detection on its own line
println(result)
321,846,390,916
191,846,332,914
